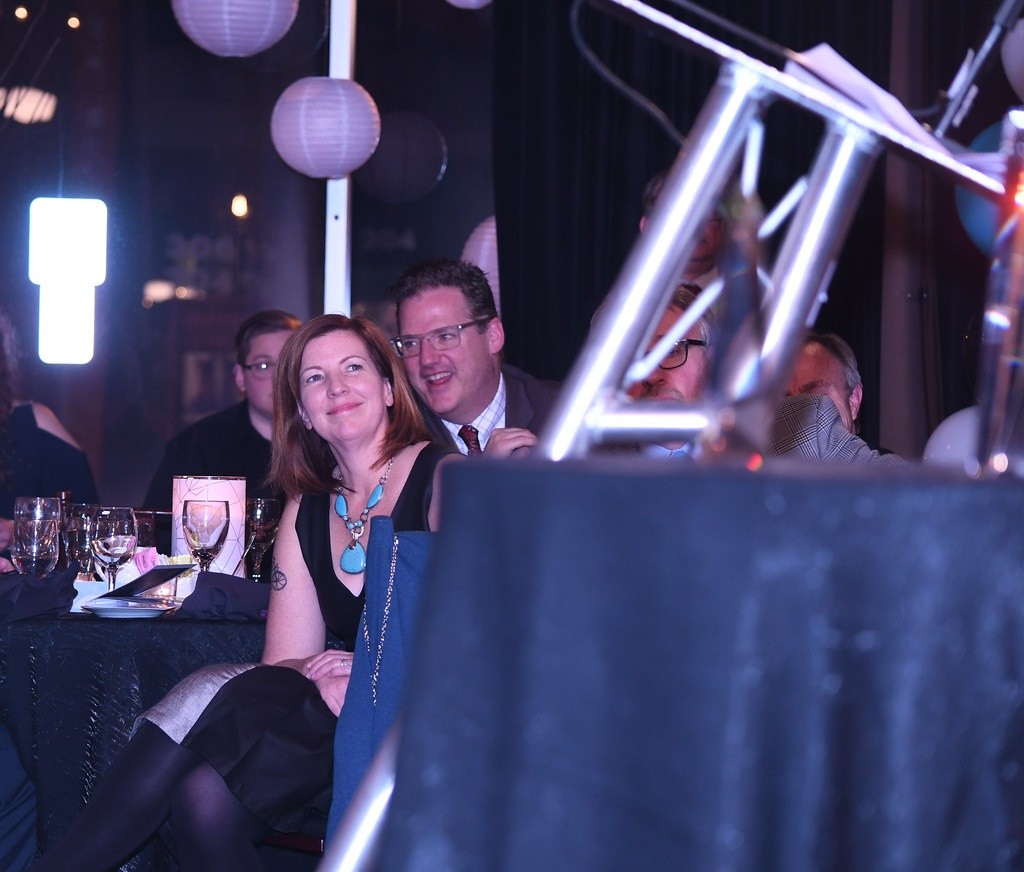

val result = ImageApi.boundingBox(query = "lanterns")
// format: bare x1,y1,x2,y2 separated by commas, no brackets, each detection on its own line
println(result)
173,0,299,58
354,108,447,205
271,77,381,179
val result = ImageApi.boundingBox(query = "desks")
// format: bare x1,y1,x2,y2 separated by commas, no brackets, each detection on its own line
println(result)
0,612,350,872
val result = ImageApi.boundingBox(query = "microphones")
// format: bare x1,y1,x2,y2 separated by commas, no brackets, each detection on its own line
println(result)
935,0,1024,138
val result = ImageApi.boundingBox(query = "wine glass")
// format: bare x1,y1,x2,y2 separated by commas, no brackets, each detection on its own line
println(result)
182,499,230,573
245,498,282,582
89,506,139,594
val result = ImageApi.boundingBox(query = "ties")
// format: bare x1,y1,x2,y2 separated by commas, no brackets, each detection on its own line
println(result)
457,424,482,457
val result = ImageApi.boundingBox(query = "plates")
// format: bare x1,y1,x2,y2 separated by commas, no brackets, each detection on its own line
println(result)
81,599,177,619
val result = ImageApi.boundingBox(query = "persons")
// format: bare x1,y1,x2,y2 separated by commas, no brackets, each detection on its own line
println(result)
587,282,911,467
41,313,471,872
782,333,896,457
0,309,101,573
639,169,769,327
384,260,555,466
145,309,305,585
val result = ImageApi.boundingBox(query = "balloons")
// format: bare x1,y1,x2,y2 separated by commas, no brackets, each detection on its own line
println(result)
950,121,1009,258
1000,16,1024,104
923,406,1024,480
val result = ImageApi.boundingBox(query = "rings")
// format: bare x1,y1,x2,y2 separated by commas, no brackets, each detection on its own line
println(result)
341,658,349,667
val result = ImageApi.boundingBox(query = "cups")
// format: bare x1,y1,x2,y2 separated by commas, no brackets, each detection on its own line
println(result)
10,497,95,577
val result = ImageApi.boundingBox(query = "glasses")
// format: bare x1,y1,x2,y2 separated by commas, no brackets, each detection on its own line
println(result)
390,315,496,358
236,361,278,380
645,339,711,370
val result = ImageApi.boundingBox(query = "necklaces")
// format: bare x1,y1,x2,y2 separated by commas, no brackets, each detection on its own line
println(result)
361,531,401,714
334,450,395,575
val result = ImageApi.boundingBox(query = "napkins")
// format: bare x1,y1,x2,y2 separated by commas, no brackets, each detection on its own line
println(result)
174,571,270,625
0,559,82,626
